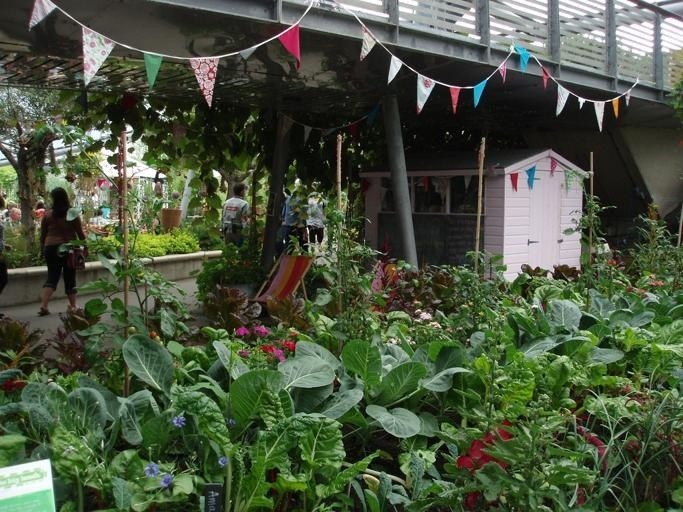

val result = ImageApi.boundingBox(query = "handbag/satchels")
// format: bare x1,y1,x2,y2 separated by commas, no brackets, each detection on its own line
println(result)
67,248,85,269
232,218,243,234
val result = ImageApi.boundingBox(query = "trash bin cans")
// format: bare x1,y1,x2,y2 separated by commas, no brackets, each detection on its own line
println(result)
162,209,183,229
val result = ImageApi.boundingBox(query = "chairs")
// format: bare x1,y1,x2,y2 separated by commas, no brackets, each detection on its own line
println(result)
246,249,317,304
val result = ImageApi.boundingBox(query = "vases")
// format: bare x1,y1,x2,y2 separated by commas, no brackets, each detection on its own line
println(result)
162,208,181,234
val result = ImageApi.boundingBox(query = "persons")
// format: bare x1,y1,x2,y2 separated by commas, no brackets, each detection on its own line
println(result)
281,193,325,254
70,192,109,227
0,223,13,314
1,193,46,223
222,184,251,247
37,187,90,317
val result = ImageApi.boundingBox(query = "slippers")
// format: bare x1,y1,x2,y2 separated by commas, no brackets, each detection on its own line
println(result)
38,311,50,314
